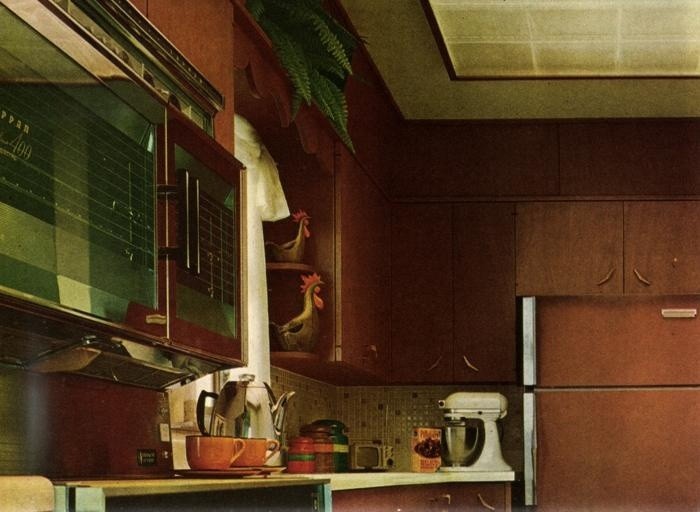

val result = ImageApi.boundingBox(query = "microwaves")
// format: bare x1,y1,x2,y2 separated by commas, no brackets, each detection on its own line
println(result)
349,443,394,470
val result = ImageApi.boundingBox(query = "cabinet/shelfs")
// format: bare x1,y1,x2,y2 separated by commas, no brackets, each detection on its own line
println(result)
277,140,384,385
516,199,700,299
158,102,249,368
390,197,516,384
326,467,516,512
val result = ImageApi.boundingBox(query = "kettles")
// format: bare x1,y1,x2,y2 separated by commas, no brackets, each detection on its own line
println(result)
199,373,295,471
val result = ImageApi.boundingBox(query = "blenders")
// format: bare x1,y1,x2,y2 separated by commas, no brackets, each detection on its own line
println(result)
438,391,513,474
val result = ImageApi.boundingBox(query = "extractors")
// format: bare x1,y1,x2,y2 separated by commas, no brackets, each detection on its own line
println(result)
34,335,191,392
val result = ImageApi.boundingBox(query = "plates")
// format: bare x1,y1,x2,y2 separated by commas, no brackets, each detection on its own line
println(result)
254,466,287,476
170,468,259,480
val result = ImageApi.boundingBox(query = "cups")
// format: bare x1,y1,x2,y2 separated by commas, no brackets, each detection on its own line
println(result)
185,432,244,470
238,436,281,468
